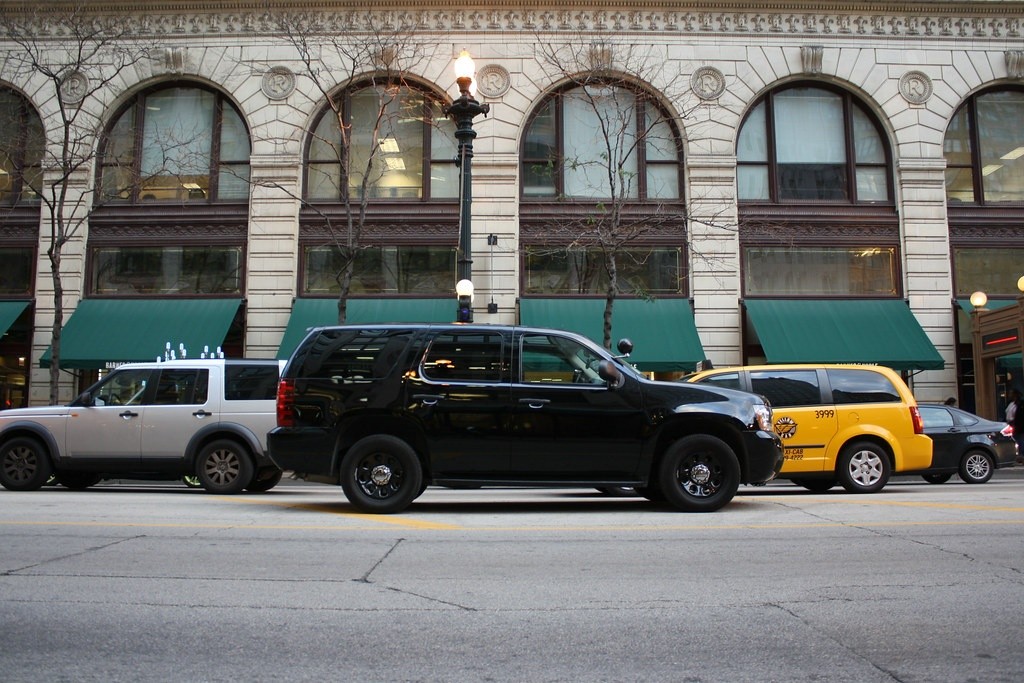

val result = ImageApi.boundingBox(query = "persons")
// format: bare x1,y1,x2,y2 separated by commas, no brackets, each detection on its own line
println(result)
945,398,955,405
1006,396,1018,422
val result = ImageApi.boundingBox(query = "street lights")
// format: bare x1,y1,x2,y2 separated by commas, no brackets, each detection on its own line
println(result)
438,48,491,323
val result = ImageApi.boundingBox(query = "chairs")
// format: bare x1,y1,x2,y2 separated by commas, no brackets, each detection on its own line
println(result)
153,377,177,406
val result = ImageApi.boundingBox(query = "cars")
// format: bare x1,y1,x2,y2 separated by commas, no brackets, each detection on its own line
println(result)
916,403,1018,484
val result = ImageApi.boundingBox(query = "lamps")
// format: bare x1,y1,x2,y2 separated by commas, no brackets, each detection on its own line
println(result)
1017,275,1024,298
969,291,987,307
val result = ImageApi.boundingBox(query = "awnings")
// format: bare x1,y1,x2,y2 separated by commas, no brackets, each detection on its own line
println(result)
957,299,1023,368
743,300,945,377
39,299,243,368
276,298,458,361
0,301,29,338
518,298,705,371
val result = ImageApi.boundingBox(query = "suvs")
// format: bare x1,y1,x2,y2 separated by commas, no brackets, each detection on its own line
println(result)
265,320,783,513
671,362,933,493
0,358,288,496
